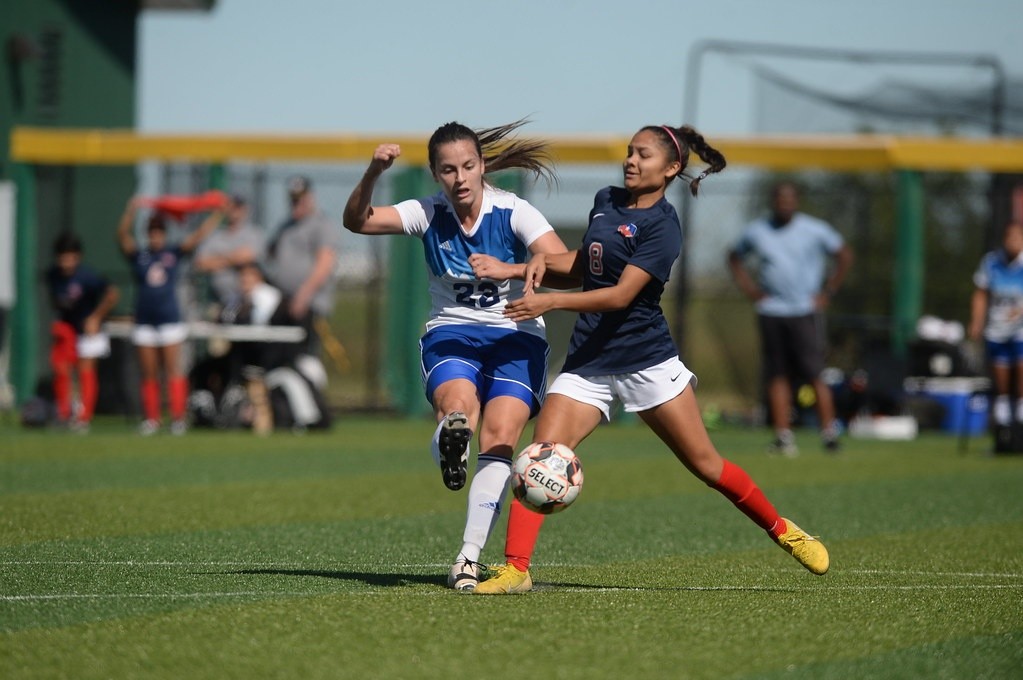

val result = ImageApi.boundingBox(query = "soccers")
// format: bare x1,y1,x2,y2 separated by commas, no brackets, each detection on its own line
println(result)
510,441,584,513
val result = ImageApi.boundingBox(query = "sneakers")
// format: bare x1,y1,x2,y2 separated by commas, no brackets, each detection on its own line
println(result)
432,410,473,490
447,553,488,592
472,564,533,596
772,518,830,574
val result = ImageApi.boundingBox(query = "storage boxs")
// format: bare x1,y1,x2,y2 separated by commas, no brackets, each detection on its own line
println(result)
904,378,991,436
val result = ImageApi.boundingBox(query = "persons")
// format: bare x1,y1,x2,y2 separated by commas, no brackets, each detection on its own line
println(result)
116,171,336,436
726,178,848,454
36,230,118,431
344,111,570,593
967,224,1023,454
471,124,828,595
